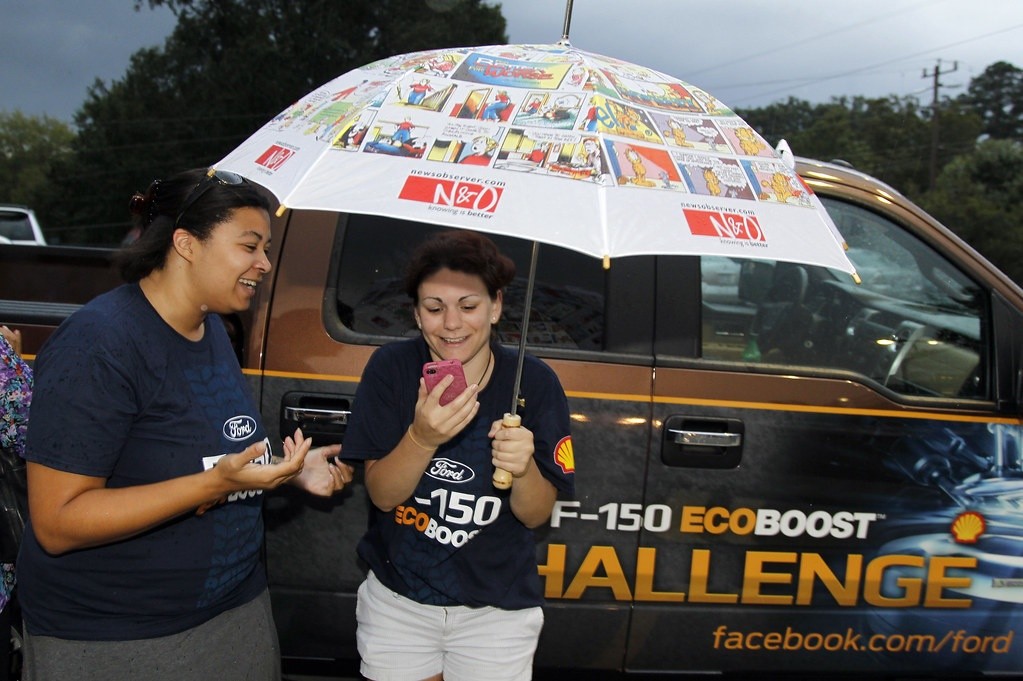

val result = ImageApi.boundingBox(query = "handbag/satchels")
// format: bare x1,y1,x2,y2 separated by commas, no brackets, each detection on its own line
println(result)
0,446,30,563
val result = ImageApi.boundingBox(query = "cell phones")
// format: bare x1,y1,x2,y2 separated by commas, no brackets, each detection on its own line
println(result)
423,359,468,407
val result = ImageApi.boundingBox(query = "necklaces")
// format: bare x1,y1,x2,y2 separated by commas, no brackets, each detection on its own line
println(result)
477,348,491,386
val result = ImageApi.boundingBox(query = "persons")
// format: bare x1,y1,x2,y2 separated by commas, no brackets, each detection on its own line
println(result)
15,167,353,681
337,229,575,681
0,324,36,681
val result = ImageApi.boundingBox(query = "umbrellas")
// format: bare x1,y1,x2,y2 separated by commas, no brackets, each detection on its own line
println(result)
205,1,860,489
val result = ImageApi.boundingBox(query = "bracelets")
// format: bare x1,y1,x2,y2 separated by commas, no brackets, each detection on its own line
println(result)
408,424,437,451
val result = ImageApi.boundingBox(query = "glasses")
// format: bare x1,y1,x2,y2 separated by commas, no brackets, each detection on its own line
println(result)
175,171,254,228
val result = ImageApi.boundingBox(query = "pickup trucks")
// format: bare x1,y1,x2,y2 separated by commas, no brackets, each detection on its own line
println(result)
0,139,1022,681
